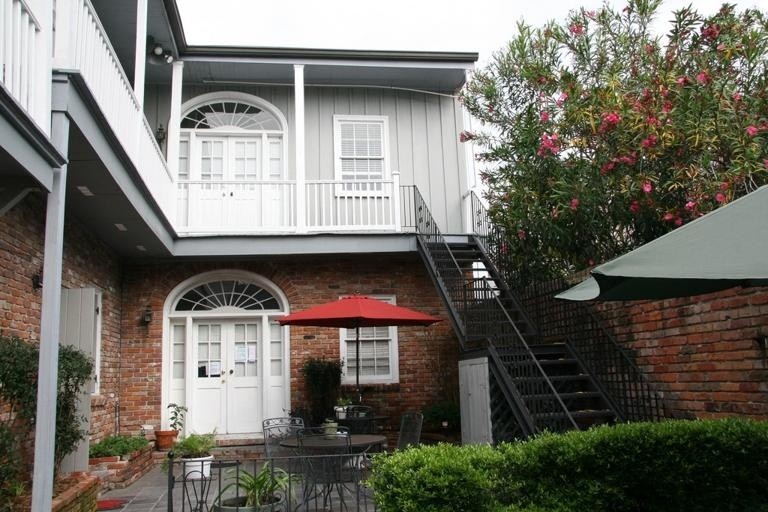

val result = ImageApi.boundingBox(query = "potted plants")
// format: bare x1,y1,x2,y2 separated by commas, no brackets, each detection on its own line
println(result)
162,432,218,481
153,404,187,450
210,461,299,512
87,434,156,491
0,328,100,512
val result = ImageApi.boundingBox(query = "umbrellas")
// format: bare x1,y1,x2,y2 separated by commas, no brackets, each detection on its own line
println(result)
274,289,444,417
553,183,768,301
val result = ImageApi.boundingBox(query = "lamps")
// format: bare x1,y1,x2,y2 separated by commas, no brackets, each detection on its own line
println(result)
141,303,153,325
32,265,42,290
150,40,176,64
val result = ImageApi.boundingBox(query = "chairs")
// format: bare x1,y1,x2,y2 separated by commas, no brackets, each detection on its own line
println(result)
260,402,425,511
